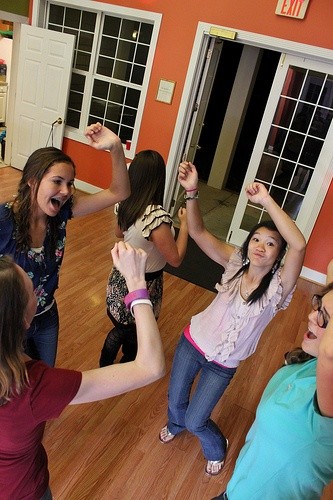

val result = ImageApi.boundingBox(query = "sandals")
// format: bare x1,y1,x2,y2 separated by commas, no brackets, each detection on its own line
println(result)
205,439,228,476
158,424,177,444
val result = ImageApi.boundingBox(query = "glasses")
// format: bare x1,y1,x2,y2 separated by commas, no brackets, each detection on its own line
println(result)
311,294,328,328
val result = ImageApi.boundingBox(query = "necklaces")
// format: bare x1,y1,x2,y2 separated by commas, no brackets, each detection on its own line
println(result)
245,279,254,296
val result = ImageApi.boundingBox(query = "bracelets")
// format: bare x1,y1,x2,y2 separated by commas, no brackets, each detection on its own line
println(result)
184,190,199,200
186,188,197,192
124,289,150,310
130,298,153,317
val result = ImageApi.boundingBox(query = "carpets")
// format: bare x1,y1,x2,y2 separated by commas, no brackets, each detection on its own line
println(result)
161,227,225,293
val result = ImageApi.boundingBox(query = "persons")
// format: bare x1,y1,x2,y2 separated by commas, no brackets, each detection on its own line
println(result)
158,160,306,476
0,241,167,500
1,121,130,369
210,259,333,500
99,149,188,366
